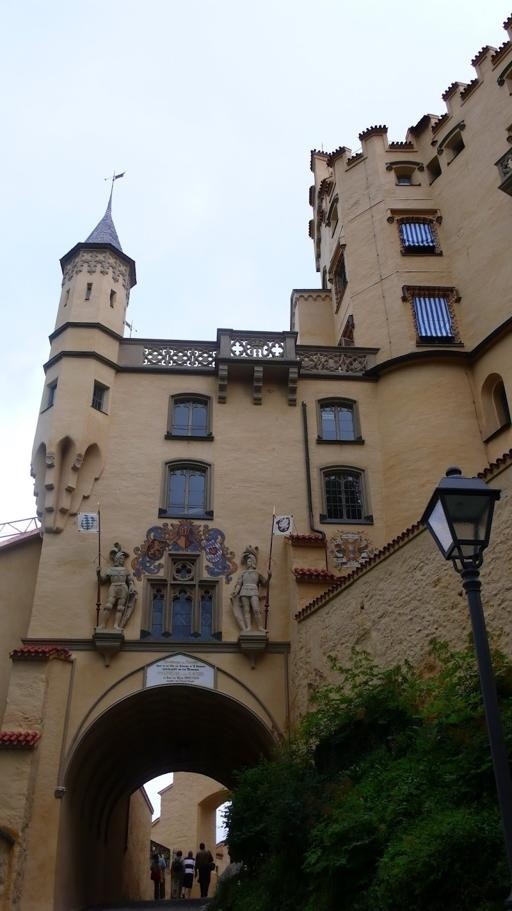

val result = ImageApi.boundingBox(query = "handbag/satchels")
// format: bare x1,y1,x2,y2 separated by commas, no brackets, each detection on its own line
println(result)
209,862,215,871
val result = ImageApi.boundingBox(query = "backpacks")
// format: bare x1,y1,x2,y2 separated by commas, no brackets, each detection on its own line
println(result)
174,858,183,873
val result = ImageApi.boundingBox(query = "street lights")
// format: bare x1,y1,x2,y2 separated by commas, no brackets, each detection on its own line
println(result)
418,463,512,863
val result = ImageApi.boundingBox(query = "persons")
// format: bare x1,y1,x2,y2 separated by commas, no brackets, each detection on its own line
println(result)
169,851,186,900
182,850,196,901
228,552,273,632
194,843,215,899
158,853,167,901
92,542,136,631
150,855,162,902
